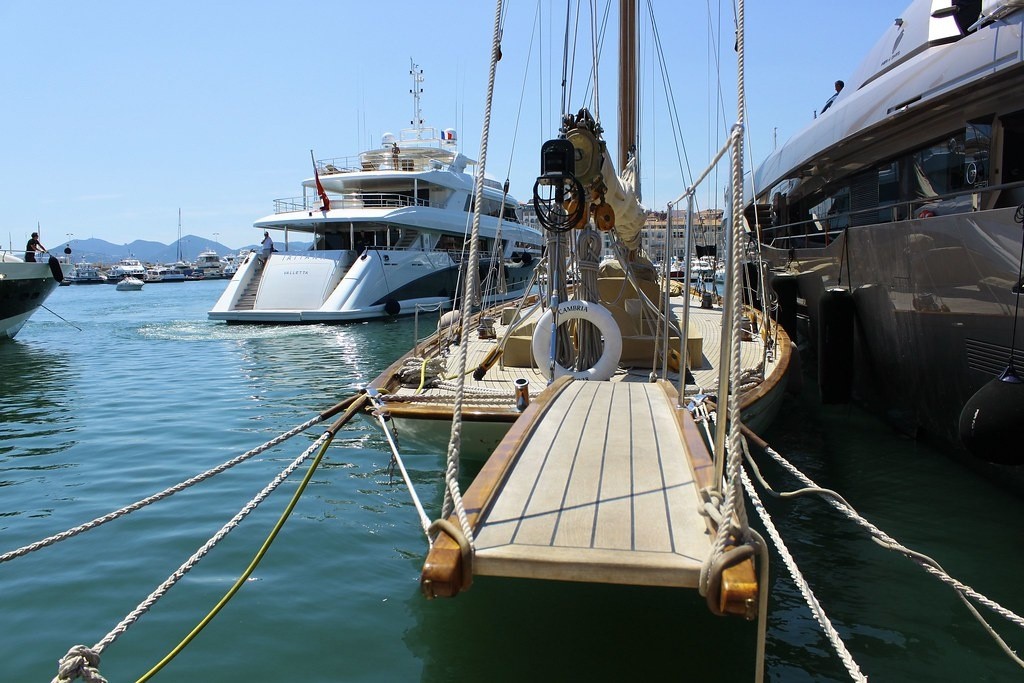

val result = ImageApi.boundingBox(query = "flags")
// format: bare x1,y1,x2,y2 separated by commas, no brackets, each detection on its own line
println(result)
315,168,329,210
696,245,717,258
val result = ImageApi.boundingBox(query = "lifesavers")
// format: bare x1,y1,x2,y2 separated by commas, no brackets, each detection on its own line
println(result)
532,299,624,382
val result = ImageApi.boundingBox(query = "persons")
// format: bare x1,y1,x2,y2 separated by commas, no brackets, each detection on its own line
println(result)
788,247,795,259
392,143,400,170
25,233,47,262
261,231,273,265
819,80,844,114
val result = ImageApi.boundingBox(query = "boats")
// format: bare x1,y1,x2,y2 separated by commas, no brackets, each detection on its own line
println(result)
116,275,145,291
0,246,73,340
208,0,1024,617
60,209,249,288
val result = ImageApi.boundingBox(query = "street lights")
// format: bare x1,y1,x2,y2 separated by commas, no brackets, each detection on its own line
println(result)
213,233,219,252
66,234,74,247
